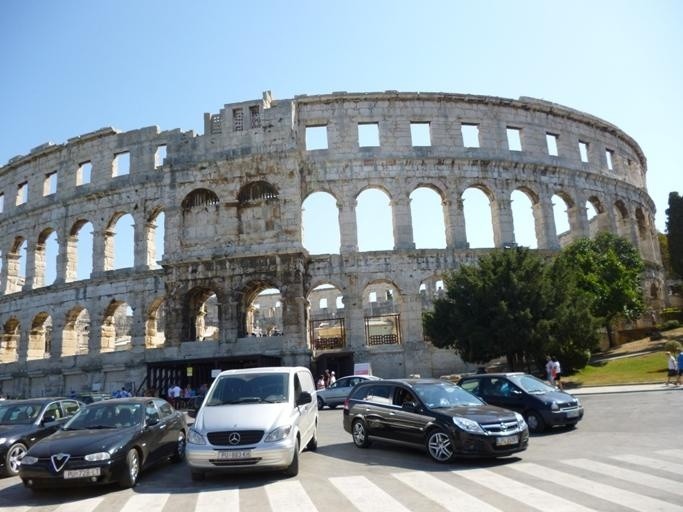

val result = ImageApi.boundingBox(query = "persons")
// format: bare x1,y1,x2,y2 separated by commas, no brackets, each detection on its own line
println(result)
324,368,331,388
671,347,683,386
70,391,77,400
545,355,554,385
663,352,682,385
400,392,417,408
550,354,563,392
112,382,209,410
328,371,337,387
316,374,326,390
244,329,285,337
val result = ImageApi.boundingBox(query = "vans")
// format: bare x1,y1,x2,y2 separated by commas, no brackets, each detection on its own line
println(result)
188,366,323,477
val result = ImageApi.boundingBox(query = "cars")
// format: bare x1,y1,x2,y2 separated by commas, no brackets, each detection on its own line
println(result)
0,397,86,479
312,371,385,414
16,394,186,491
451,369,586,437
342,377,533,468
